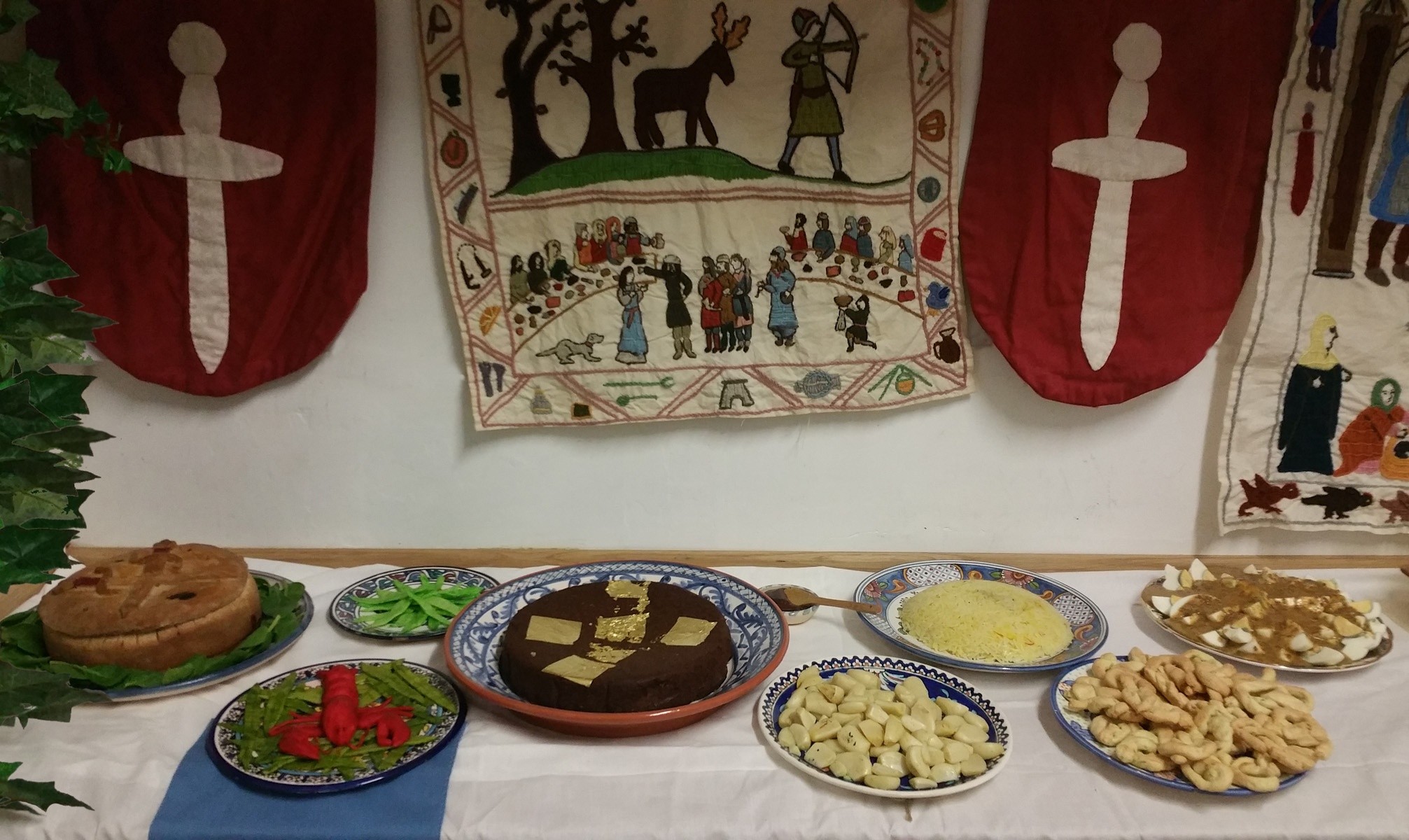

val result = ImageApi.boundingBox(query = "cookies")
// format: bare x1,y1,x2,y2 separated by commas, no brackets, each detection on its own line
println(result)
1059,646,1333,792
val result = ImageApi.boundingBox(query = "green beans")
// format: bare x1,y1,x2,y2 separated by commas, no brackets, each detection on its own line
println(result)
218,657,458,783
345,573,485,634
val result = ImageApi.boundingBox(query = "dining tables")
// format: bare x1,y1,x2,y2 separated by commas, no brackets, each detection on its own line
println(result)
2,556,1409,840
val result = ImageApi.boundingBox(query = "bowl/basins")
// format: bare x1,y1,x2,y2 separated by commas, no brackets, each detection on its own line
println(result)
757,584,820,627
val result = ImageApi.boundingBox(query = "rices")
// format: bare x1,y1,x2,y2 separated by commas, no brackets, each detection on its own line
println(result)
900,579,1074,665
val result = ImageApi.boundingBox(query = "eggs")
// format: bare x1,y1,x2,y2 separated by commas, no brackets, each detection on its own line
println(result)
1152,558,1391,666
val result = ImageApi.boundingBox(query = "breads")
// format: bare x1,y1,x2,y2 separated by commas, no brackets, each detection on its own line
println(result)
39,540,261,669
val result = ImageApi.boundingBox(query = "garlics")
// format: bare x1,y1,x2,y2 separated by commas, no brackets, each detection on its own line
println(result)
778,664,1004,794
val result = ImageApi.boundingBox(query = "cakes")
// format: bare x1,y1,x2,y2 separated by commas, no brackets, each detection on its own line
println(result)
499,579,738,713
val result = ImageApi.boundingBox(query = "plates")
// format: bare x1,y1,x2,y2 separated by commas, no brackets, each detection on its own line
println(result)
443,558,790,736
1050,653,1318,797
1143,564,1394,674
761,654,1014,800
854,559,1109,674
328,566,503,642
10,567,316,704
211,657,467,792
